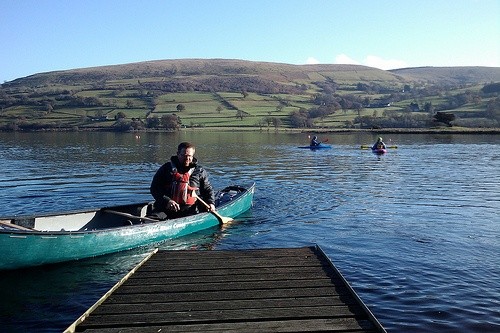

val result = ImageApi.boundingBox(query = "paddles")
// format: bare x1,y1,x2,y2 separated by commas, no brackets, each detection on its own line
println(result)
321,139,328,143
191,190,234,223
361,146,398,150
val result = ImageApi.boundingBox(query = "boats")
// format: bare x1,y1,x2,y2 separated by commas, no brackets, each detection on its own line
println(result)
372,149,387,154
0,181,256,273
297,143,332,149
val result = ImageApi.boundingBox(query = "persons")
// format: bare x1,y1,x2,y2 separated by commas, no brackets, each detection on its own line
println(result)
372,137,386,150
145,143,216,221
310,136,318,146
